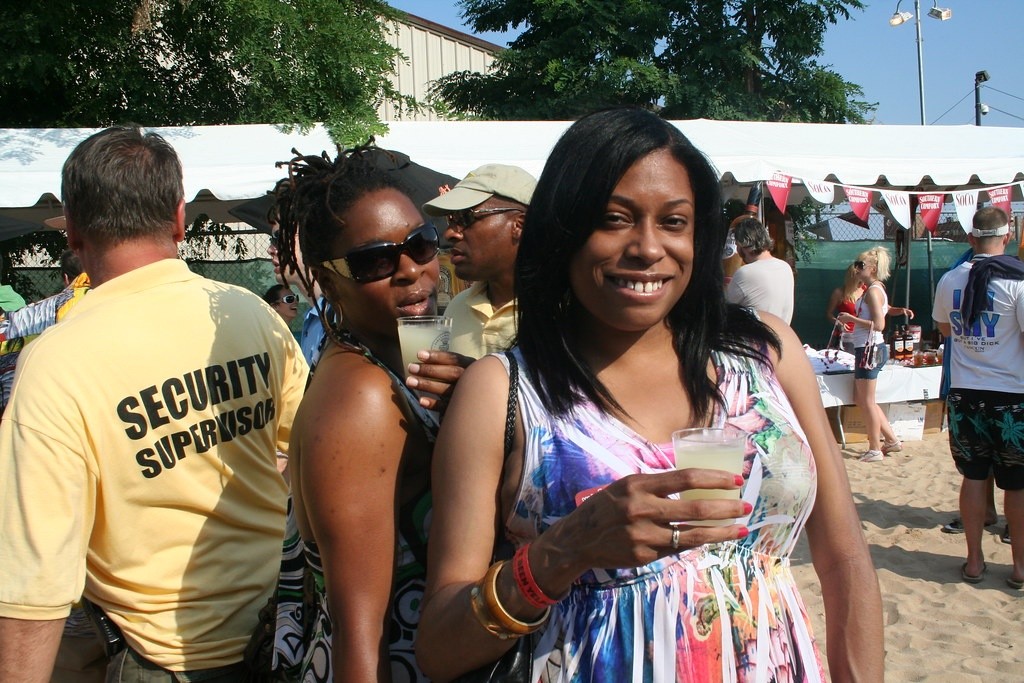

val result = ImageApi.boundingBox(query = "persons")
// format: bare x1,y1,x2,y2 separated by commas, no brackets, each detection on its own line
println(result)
827,246,914,462
417,107,884,683
0,123,538,683
723,217,795,326
932,207,1024,588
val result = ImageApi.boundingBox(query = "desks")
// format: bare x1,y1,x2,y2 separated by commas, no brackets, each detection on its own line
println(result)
816,362,943,450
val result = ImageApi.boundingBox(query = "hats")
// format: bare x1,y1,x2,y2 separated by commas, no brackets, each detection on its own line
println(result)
421,163,538,218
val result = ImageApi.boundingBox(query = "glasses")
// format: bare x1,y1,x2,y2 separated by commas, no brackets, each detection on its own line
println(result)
315,220,440,284
854,261,864,270
446,208,524,233
276,294,299,304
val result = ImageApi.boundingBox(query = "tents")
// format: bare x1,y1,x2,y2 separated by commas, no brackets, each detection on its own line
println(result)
0,119,1024,328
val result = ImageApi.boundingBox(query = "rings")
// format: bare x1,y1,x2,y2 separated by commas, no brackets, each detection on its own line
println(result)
673,524,679,549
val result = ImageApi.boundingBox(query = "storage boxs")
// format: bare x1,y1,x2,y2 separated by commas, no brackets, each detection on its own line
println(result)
826,400,945,444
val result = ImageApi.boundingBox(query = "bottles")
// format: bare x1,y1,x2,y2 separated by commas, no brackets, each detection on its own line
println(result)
902,326,914,359
890,324,904,362
765,192,797,279
720,187,762,293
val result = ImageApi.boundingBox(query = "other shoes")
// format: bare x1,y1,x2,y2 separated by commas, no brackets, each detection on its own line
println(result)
1003,525,1011,543
944,518,990,533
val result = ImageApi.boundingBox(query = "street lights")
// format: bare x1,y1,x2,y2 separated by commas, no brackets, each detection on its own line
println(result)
887,0,954,350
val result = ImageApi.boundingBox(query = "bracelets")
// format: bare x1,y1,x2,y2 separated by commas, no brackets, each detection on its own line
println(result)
470,541,561,639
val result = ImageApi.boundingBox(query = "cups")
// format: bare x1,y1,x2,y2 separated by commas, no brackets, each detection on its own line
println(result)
396,314,453,387
924,352,937,365
913,351,923,367
936,349,944,364
673,426,743,539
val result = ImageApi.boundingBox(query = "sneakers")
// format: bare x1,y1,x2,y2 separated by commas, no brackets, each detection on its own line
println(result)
881,440,902,454
858,449,883,462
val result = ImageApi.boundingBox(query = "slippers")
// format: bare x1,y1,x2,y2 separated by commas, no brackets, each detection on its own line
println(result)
1007,579,1024,588
961,562,986,583
